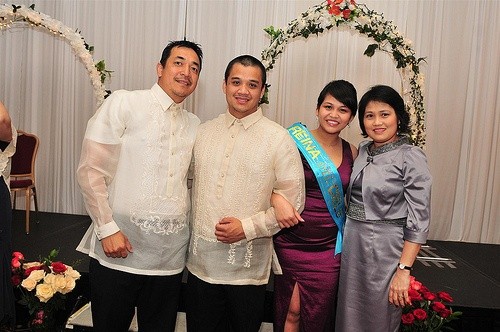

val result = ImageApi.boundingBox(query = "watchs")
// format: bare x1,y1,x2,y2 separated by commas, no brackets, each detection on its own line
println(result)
398,263,411,270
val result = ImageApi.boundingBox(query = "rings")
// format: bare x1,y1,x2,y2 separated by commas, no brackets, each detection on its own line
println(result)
399,295,403,301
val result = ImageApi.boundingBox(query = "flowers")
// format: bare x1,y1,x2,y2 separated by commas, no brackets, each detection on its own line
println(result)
0,4,111,108
261,0,427,149
402,276,461,332
12,249,82,332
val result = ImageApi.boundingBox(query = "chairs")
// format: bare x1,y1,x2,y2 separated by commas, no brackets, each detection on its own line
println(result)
9,130,39,234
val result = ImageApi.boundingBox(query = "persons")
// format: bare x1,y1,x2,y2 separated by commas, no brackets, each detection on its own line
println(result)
186,55,306,332
272,80,357,332
76,40,203,332
0,101,17,332
335,85,432,332
